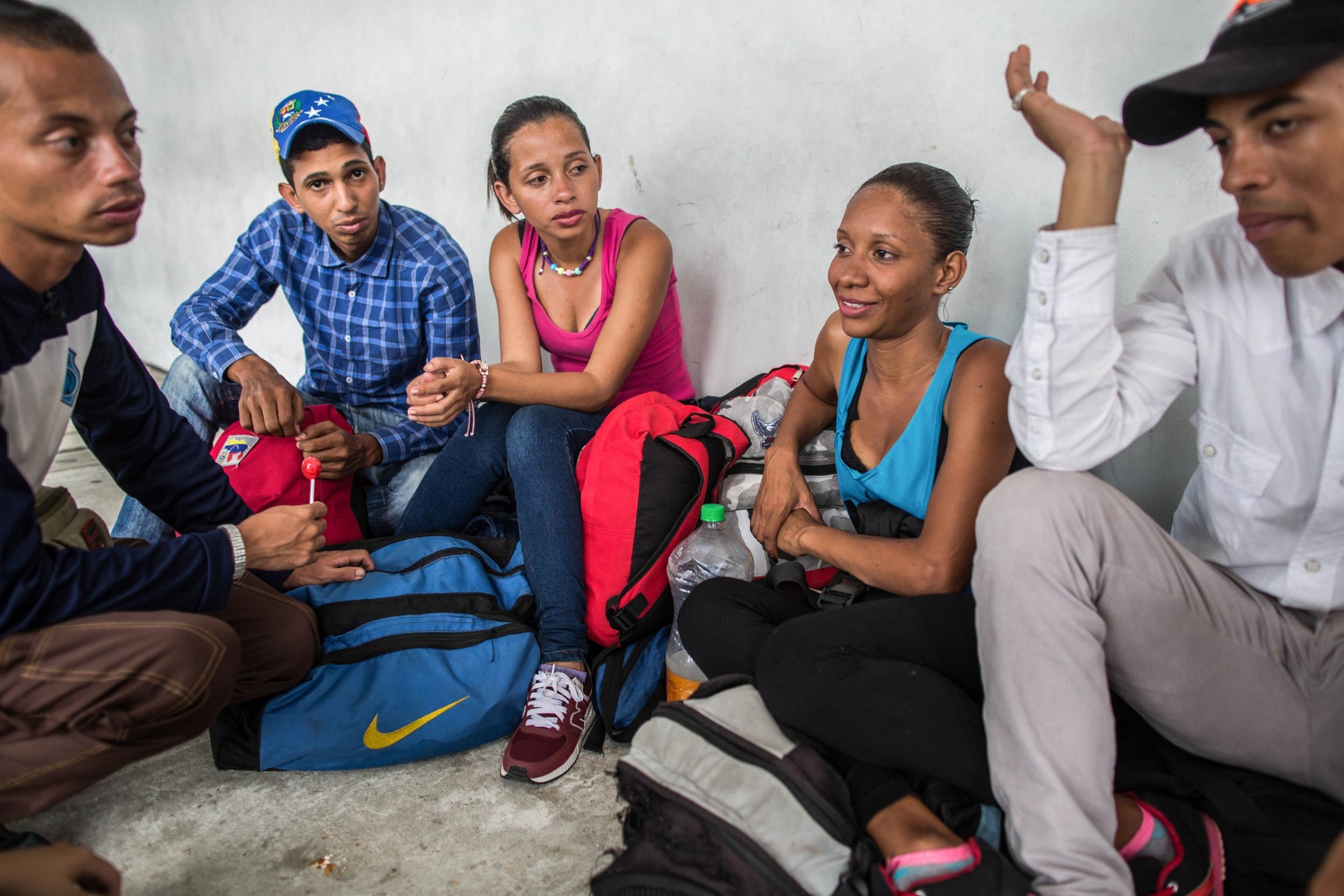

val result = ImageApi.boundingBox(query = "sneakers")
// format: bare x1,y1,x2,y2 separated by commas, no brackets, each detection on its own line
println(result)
500,659,598,783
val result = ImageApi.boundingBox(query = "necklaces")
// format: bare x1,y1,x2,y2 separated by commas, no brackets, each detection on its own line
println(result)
538,207,601,277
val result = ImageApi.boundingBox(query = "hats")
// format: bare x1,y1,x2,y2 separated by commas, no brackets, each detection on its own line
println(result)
1123,0,1344,146
270,90,370,174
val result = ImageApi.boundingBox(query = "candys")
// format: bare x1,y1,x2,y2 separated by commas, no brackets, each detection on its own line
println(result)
301,456,322,480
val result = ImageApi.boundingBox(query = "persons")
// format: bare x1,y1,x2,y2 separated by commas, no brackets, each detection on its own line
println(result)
0,0,376,896
113,92,479,547
971,1,1344,896
678,162,1230,896
397,96,696,780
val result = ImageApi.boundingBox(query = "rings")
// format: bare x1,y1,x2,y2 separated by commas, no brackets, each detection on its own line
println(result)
1013,88,1036,112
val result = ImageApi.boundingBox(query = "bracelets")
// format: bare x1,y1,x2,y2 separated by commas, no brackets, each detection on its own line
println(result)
464,358,490,437
216,524,247,582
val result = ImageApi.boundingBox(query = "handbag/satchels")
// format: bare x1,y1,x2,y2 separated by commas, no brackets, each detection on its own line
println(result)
766,501,924,607
712,364,843,511
209,404,371,547
210,515,541,770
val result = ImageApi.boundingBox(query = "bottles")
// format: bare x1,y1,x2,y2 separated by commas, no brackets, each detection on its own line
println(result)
665,502,755,702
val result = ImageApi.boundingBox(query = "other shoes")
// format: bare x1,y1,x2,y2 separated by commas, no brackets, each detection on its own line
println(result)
1120,791,1226,896
873,837,1035,896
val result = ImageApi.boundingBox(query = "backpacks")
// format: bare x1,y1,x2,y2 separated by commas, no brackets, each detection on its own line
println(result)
590,675,872,896
574,392,751,647
